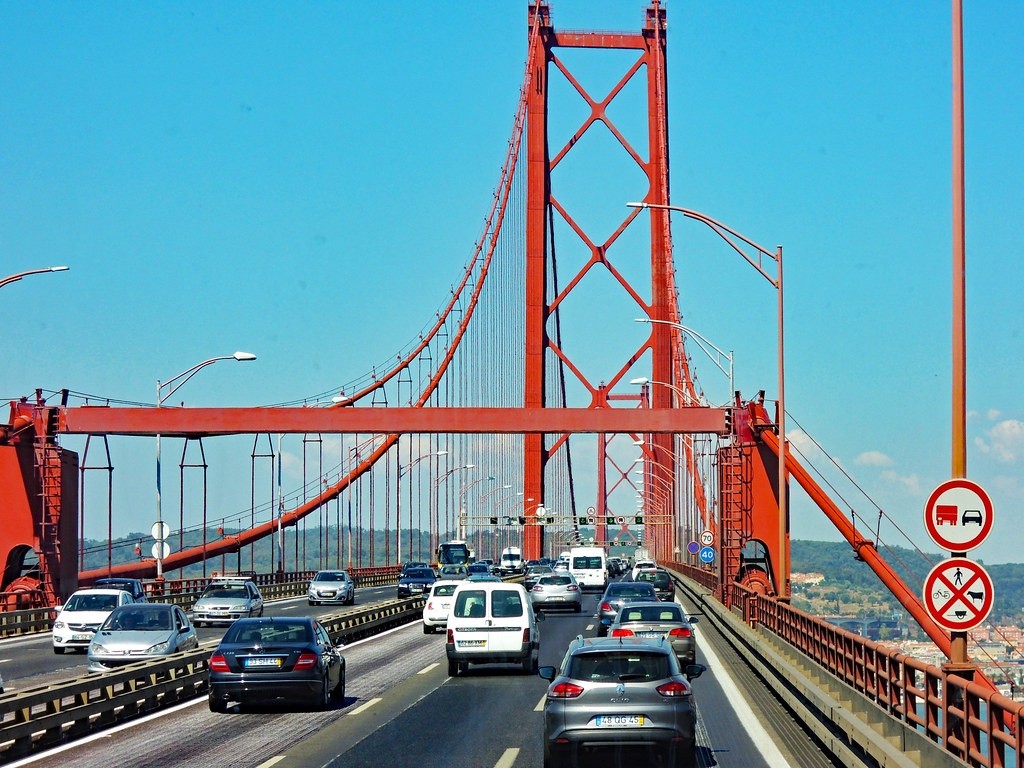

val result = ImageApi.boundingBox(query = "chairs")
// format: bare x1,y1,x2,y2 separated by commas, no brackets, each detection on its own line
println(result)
659,575,666,580
468,604,484,617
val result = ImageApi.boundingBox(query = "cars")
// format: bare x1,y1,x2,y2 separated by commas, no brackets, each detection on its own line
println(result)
54,588,137,655
189,581,263,627
398,568,441,600
209,616,346,712
524,552,570,593
92,578,150,603
597,562,700,665
397,561,429,581
606,556,631,578
531,571,583,613
540,634,707,767
87,603,199,673
467,559,504,582
307,570,354,605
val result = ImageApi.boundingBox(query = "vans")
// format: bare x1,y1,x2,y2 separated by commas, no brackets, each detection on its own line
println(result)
446,581,546,674
569,547,610,593
501,546,525,575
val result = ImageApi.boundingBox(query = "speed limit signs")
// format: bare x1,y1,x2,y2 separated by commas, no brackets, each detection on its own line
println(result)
699,547,715,564
699,530,714,546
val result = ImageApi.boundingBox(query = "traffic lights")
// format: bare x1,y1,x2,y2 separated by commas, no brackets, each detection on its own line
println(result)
579,517,586,525
607,517,614,525
635,517,642,524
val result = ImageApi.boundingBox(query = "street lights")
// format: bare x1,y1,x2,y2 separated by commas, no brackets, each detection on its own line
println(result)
274,397,356,572
633,317,734,443
399,451,447,566
156,351,256,602
635,438,720,562
627,201,794,603
434,465,588,561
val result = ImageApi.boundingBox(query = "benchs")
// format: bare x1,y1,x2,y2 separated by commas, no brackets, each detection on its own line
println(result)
628,612,673,620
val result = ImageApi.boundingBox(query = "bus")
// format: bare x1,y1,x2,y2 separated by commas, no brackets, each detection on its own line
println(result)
435,540,476,573
